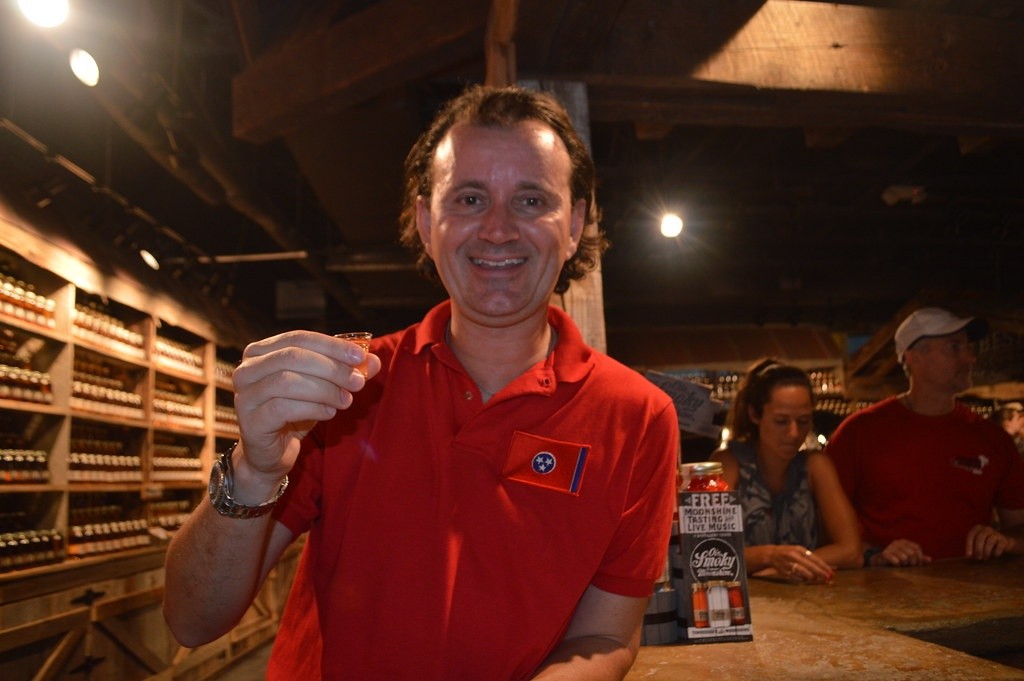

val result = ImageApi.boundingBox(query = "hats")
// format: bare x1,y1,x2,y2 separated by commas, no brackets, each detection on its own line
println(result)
894,307,974,363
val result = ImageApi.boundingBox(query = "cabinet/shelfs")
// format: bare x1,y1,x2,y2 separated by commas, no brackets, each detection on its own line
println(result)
0,219,305,681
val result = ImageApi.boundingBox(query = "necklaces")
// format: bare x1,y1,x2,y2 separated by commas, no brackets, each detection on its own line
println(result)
446,318,556,396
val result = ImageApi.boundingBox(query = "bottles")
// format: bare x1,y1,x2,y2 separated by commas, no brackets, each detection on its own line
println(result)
679,462,729,492
727,581,746,625
0,275,240,572
707,581,731,627
691,583,709,628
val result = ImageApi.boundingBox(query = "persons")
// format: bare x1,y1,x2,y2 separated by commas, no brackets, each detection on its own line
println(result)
823,307,1024,570
164,87,678,681
704,357,863,582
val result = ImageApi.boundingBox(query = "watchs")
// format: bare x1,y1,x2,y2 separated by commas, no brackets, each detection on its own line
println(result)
208,442,288,520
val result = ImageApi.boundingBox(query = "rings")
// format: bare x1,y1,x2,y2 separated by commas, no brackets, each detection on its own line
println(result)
804,549,812,558
786,571,791,580
792,563,798,574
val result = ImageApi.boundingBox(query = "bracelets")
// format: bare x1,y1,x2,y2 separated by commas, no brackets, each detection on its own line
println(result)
864,545,885,568
